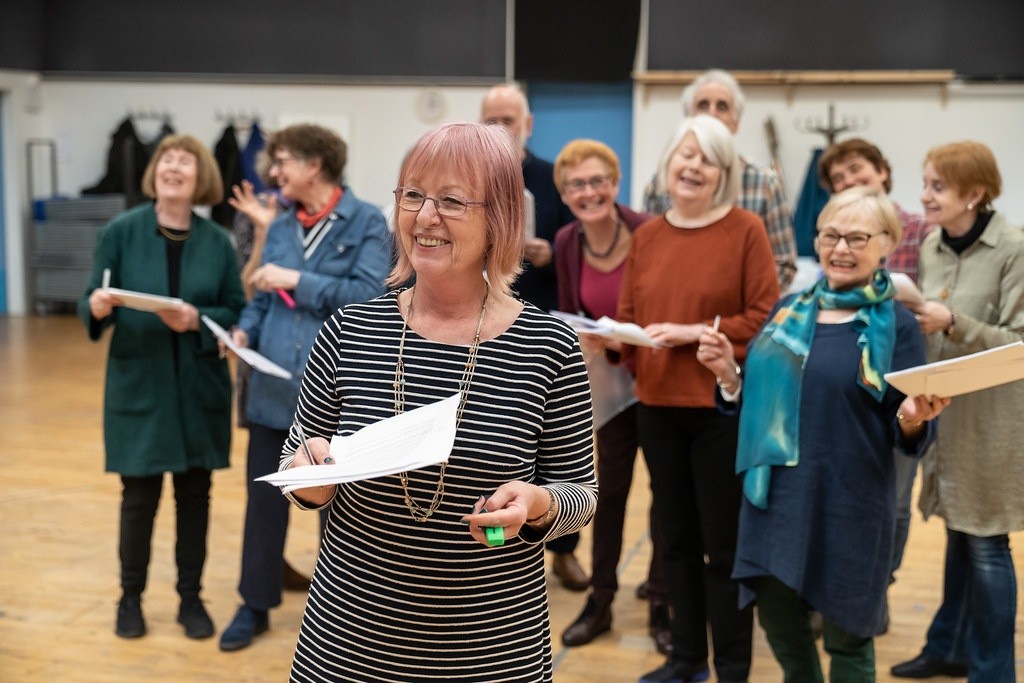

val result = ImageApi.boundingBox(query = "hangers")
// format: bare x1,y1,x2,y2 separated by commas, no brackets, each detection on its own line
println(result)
128,93,169,122
234,123,269,138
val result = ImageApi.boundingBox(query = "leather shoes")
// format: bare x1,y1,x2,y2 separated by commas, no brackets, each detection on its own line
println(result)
647,622,673,653
562,604,613,645
891,651,968,678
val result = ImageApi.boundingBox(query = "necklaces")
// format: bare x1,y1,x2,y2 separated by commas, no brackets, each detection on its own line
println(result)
159,225,189,241
392,280,489,524
581,206,621,259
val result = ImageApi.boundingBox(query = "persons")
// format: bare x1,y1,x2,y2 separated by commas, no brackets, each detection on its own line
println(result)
275,122,598,683
76,126,389,654
481,67,1024,683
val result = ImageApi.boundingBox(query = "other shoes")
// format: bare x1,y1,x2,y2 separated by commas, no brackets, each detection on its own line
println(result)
177,597,215,637
552,550,588,589
218,604,271,650
279,559,311,590
808,608,824,640
638,578,652,598
115,594,146,638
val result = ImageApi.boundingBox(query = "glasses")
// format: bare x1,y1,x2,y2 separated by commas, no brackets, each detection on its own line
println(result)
561,173,617,195
267,157,309,172
393,184,486,217
818,226,892,250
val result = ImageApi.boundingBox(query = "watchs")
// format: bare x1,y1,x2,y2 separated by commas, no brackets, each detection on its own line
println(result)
944,314,956,336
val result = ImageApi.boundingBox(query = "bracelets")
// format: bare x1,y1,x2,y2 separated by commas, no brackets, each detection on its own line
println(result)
525,489,556,529
895,412,921,428
717,362,742,390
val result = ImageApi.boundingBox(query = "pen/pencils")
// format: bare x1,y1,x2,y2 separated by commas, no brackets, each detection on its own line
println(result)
712,314,721,333
277,287,295,309
102,268,111,288
293,418,316,465
